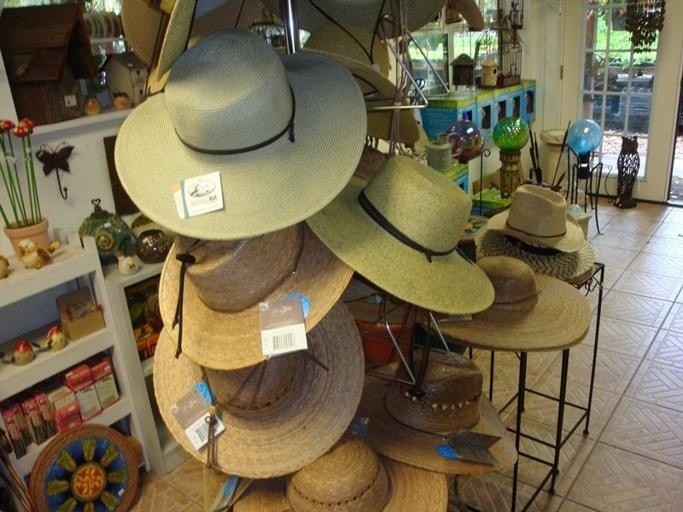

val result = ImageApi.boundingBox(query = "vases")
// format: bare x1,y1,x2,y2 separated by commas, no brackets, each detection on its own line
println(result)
2,217,49,258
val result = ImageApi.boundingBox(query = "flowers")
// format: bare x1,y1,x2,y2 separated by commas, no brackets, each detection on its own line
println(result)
0,114,42,228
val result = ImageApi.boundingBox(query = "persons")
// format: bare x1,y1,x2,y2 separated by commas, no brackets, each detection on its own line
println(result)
593,64,623,113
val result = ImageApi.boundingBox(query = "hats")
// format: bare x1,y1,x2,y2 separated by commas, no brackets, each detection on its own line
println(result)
474,183,595,287
114,1,517,512
425,255,591,352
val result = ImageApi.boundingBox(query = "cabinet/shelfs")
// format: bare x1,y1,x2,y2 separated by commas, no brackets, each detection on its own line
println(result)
104,260,190,477
0,235,160,512
